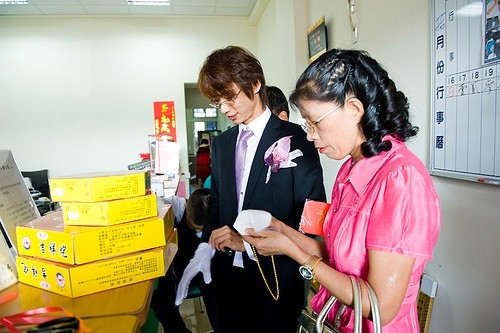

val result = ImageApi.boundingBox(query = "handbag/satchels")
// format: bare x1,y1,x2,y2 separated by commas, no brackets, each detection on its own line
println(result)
292,276,382,333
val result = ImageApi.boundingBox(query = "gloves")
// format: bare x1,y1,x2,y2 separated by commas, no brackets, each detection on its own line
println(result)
175,243,215,306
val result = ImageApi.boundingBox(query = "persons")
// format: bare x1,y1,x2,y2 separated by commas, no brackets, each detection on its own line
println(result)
195,139,212,188
264,86,289,122
241,48,440,333
175,45,327,333
151,188,210,333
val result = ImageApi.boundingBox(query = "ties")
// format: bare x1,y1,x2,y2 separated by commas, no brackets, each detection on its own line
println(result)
234,128,254,204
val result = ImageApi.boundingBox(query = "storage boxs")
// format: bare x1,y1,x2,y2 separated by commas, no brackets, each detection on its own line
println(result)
15,169,178,298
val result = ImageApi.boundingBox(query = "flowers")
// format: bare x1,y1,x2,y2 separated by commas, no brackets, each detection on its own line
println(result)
265,136,303,184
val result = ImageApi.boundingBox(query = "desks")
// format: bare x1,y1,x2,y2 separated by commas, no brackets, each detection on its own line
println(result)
0,280,156,333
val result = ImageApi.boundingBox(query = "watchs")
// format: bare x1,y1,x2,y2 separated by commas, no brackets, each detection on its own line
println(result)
299,255,321,280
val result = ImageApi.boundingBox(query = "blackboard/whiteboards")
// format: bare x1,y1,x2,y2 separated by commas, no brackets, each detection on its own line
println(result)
430,0,500,185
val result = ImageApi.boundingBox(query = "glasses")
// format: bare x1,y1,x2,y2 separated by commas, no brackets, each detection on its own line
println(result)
300,97,353,134
208,88,243,108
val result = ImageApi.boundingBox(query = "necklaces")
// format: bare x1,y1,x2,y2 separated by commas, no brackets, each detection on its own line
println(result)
251,244,279,301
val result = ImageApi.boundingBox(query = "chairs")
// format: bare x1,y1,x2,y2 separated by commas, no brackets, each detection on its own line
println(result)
20,169,54,212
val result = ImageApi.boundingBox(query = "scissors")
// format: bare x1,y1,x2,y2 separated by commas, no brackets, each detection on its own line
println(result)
21,317,79,333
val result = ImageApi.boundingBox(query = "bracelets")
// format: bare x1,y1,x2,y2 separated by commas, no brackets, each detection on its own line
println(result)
310,257,322,282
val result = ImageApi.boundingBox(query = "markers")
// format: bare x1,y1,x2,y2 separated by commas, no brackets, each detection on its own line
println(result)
477,178,500,185
